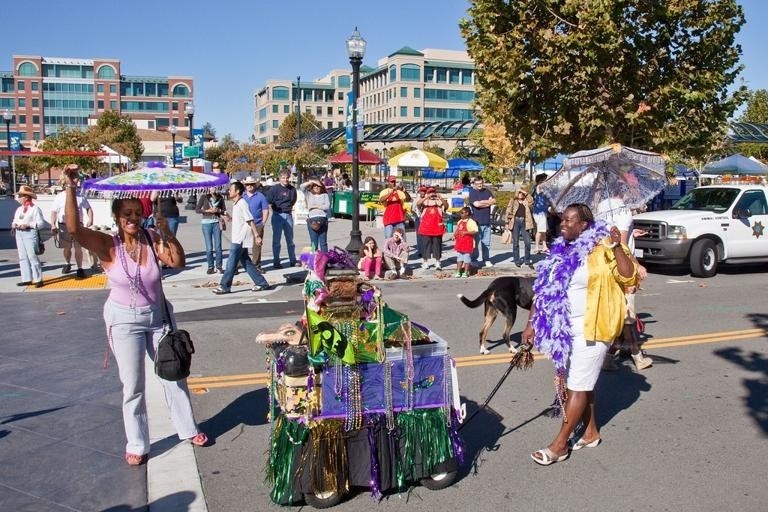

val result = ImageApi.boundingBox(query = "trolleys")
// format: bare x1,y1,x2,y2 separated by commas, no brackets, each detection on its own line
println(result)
85,230,115,269
258,319,533,510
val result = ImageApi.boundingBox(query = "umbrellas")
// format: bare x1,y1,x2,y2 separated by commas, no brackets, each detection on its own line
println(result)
329,148,385,178
79,160,230,253
535,144,669,247
387,150,449,193
447,158,484,184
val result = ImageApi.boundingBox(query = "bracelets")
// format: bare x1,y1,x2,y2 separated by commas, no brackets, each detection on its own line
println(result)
528,319,534,321
64,181,76,190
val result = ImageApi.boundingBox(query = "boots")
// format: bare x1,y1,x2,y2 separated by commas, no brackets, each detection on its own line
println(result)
630,350,653,370
603,353,620,371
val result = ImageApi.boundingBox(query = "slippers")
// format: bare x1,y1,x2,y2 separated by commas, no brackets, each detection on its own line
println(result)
192,432,208,445
126,452,145,465
530,447,568,466
567,436,600,450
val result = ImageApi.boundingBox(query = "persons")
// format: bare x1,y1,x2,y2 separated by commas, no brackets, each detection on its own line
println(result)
452,172,496,278
136,189,182,236
62,163,210,463
195,171,352,274
12,174,93,288
357,228,412,279
211,181,268,293
593,180,653,372
379,175,447,269
522,204,639,464
505,173,553,267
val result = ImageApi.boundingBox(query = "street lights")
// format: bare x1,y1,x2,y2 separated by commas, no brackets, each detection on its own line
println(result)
0,107,16,197
169,123,180,168
185,97,196,170
45,127,52,187
343,22,368,255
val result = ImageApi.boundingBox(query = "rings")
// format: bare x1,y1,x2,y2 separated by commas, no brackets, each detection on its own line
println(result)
614,233,619,238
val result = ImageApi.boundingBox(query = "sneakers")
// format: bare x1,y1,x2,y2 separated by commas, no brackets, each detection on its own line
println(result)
471,262,480,268
423,263,430,269
483,260,493,268
62,264,71,273
436,264,442,270
462,273,468,278
207,268,214,274
291,262,300,267
77,268,83,277
455,272,461,278
216,268,223,274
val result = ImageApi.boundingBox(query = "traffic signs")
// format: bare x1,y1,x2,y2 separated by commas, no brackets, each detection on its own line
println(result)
182,144,199,158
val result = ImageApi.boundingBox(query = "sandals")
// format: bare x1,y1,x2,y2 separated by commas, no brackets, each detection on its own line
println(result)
252,284,269,291
213,287,228,293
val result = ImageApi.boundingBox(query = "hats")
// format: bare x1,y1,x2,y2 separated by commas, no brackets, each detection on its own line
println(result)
388,176,396,182
243,177,260,188
12,186,37,199
419,187,436,193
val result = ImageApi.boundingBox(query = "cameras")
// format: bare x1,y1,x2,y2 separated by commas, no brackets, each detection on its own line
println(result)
431,195,437,200
397,186,403,191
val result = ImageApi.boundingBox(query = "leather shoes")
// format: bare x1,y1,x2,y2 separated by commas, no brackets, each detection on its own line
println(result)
17,282,31,286
36,281,43,287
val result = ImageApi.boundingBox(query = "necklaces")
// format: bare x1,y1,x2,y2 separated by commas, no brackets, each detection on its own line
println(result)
115,232,142,307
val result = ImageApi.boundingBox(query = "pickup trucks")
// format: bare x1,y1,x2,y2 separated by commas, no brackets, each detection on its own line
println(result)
630,179,768,281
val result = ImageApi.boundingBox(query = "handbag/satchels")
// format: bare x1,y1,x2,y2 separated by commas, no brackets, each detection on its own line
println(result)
157,330,195,381
218,217,226,230
500,230,512,244
35,239,45,255
308,219,325,232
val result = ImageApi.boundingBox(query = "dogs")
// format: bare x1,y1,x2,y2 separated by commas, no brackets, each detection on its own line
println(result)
456,276,538,355
